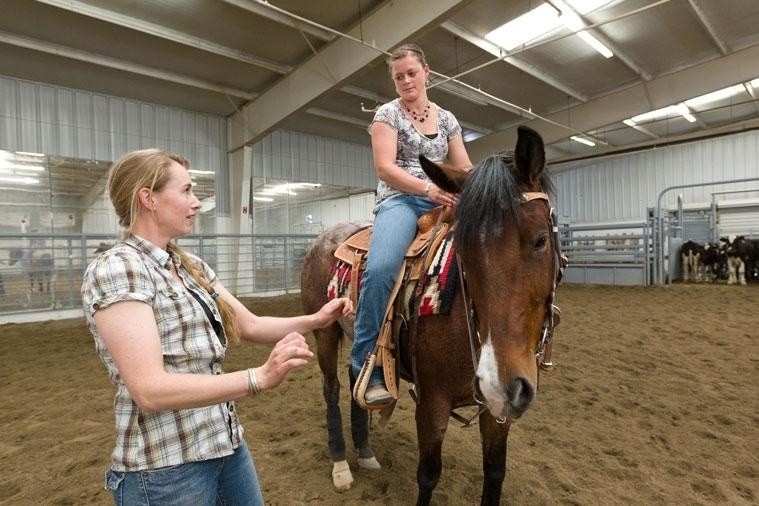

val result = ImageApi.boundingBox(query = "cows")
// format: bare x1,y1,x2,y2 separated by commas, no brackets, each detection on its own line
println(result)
680,235,759,286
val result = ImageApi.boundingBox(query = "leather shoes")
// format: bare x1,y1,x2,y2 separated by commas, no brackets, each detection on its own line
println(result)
364,384,393,406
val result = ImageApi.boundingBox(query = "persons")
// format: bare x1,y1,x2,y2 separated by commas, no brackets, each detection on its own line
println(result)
79,148,354,506
348,43,474,406
21,228,47,274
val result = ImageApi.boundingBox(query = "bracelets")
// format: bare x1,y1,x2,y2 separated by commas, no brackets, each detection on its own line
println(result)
246,366,262,397
424,183,431,196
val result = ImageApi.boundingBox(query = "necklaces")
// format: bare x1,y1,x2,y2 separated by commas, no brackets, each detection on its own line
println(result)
402,101,430,123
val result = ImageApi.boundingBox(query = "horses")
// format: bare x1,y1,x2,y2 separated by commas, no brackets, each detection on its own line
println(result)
300,126,557,506
